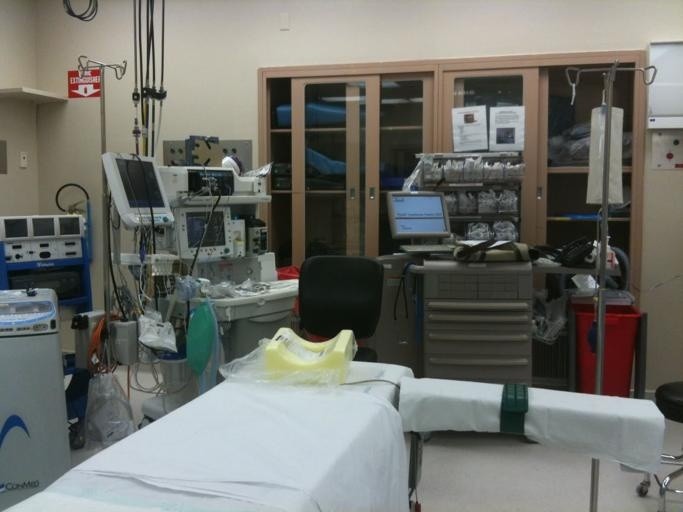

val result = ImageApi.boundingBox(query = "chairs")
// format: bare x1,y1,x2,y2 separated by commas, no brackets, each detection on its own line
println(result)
299,255,384,361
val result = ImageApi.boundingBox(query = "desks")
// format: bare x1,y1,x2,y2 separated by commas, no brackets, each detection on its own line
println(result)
173,278,300,360
394,373,667,512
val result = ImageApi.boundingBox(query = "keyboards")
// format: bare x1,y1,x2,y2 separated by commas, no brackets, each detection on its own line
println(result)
399,245,454,254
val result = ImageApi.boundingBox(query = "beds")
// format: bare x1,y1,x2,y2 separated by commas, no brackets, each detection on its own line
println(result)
0,359,424,512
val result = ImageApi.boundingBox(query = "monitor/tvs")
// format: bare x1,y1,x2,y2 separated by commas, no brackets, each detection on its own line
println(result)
175,206,234,259
386,190,451,244
101,152,175,227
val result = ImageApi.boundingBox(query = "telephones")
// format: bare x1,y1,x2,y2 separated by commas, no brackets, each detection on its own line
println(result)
553,237,594,267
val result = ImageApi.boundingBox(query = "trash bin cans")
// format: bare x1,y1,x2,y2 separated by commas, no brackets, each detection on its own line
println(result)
568,287,642,398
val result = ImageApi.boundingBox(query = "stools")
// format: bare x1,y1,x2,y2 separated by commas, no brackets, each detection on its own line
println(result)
634,380,683,512
